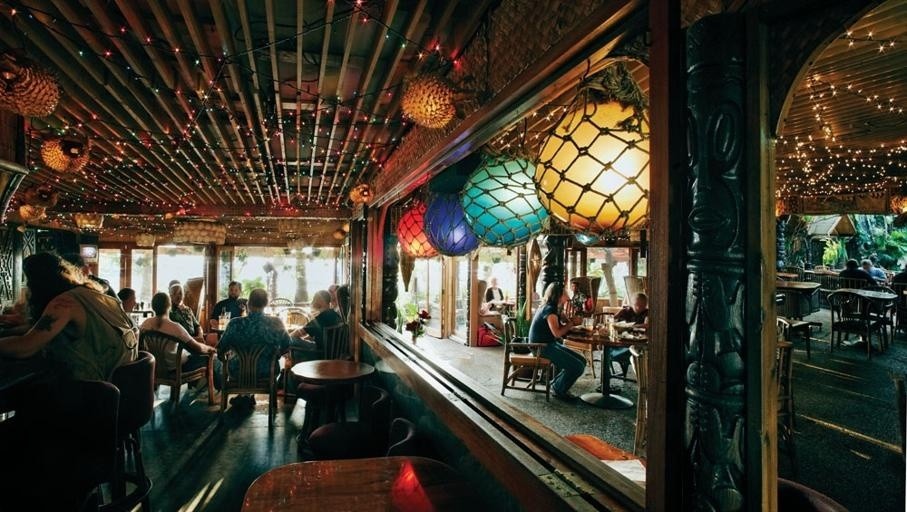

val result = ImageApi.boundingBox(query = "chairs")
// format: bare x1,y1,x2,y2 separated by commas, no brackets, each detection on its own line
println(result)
123,287,355,427
563,339,597,380
500,317,558,400
777,314,800,479
627,342,648,456
298,382,422,457
622,274,649,306
570,275,601,315
0,350,157,511
239,456,495,511
777,263,907,362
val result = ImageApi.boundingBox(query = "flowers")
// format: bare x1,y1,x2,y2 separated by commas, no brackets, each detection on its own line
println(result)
405,310,432,337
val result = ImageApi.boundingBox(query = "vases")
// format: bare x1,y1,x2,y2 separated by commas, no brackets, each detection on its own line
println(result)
412,335,422,349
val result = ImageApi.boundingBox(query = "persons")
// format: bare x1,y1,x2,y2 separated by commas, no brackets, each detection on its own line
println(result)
486,278,503,312
838,258,878,296
889,264,906,334
276,284,342,390
854,259,886,283
569,282,587,311
116,280,222,403
526,281,587,402
217,288,292,409
1,252,137,385
209,281,248,341
614,292,650,325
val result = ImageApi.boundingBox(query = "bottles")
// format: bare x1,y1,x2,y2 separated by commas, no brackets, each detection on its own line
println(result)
889,273,893,282
0,299,18,329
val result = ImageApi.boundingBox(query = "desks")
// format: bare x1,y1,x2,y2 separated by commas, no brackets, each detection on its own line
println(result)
289,358,378,381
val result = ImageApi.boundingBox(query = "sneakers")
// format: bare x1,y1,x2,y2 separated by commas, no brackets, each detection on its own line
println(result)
230,395,256,407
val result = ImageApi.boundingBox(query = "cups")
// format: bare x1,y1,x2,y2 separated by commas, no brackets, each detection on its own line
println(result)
580,312,619,342
216,307,231,328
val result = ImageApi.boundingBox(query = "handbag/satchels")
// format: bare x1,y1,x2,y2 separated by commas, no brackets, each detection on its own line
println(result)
478,323,504,347
513,338,530,353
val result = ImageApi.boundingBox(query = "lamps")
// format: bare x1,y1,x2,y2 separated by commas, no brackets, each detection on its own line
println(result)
394,74,455,128
39,137,90,176
